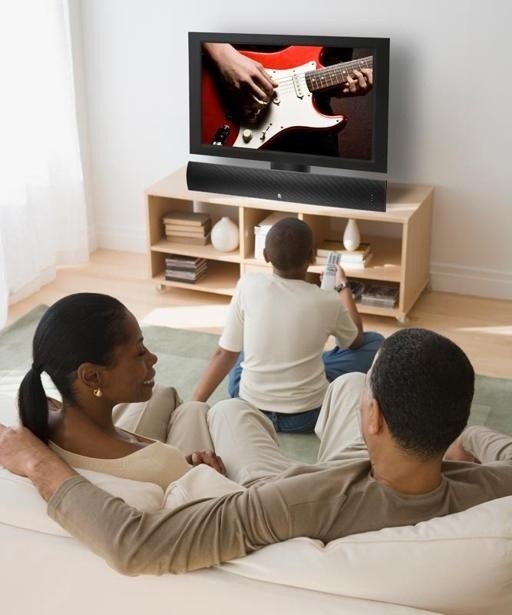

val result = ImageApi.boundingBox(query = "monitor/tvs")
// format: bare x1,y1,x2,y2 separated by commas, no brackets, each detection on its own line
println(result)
315,278,369,302
361,283,400,311
162,209,212,246
163,255,209,284
315,238,375,272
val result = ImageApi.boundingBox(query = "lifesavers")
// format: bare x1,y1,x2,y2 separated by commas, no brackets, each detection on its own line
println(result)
187,162,387,212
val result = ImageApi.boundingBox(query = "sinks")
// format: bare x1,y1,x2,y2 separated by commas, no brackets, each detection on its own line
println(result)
0,375,512,615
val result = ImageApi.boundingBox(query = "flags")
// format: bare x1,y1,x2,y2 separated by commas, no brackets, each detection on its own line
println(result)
211,216,240,251
343,218,361,250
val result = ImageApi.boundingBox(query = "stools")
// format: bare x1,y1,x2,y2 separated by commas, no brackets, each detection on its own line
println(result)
260,410,306,416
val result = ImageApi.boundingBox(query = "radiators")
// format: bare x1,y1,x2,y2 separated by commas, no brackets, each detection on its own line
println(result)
320,252,345,292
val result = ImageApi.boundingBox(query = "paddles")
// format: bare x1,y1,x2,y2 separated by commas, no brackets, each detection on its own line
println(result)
0,302,512,465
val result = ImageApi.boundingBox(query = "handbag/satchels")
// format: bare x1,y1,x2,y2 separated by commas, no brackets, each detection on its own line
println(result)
334,282,350,293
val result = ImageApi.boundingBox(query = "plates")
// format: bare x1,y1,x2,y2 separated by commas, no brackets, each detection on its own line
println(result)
143,166,433,323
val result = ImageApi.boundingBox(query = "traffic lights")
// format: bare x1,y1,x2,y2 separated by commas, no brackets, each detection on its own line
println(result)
162,464,512,615
1,464,162,539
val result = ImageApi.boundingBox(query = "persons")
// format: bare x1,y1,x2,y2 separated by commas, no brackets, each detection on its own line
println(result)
17,290,228,497
0,327,511,578
190,216,386,435
201,41,375,155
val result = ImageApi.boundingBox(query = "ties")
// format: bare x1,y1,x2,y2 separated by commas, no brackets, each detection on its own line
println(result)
189,32,390,174
